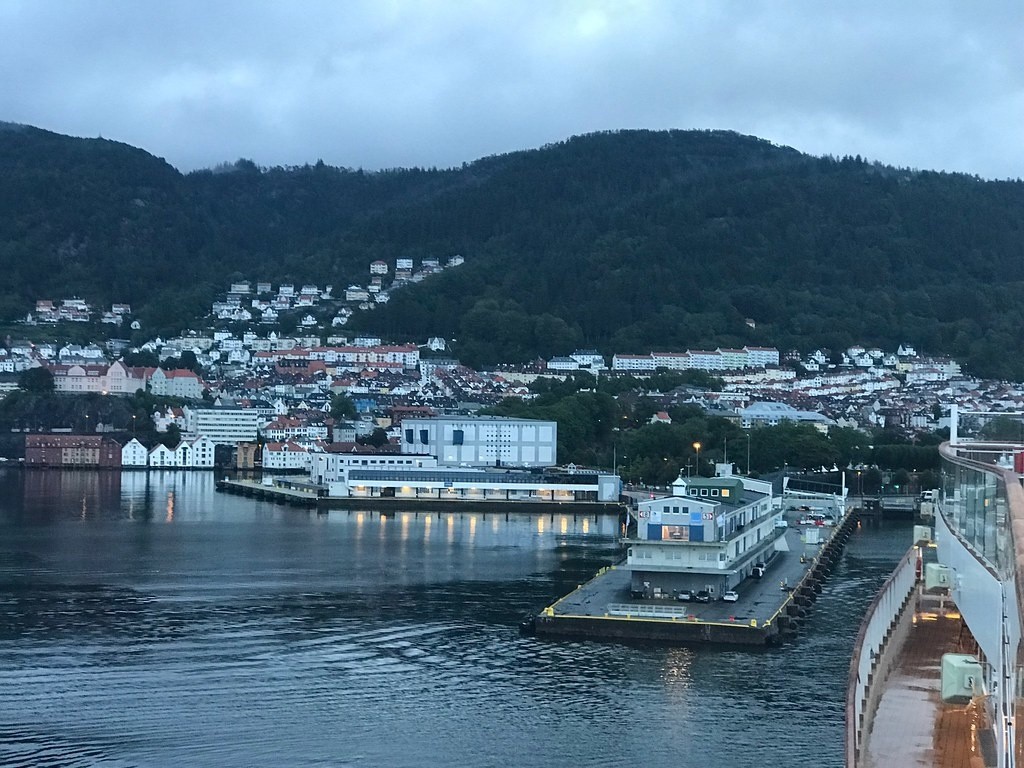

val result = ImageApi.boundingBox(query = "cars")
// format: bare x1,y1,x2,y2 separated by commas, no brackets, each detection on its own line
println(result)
678,591,693,602
723,591,739,602
695,590,711,603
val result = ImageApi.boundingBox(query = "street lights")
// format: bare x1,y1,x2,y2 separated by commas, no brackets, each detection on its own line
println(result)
857,472,861,495
785,463,788,476
747,433,750,473
695,443,700,475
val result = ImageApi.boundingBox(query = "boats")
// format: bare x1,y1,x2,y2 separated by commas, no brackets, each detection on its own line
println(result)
845,405,1024,768
